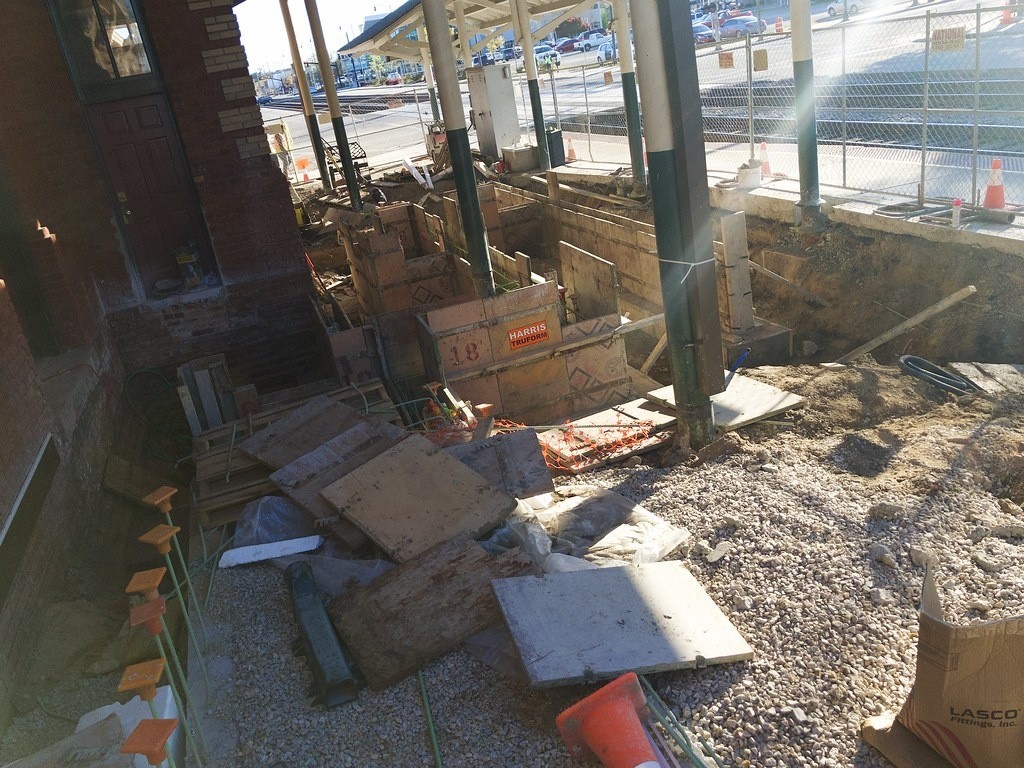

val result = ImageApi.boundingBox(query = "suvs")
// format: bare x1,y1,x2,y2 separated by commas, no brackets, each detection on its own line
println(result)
275,85,293,95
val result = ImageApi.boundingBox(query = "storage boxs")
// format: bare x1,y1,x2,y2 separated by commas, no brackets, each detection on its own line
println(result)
896,562,1024,768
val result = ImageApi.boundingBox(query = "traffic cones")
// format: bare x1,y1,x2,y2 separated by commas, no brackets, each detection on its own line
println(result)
760,142,771,176
568,136,576,159
302,165,309,181
1000,3,1014,24
982,159,1006,209
642,137,648,166
555,672,661,768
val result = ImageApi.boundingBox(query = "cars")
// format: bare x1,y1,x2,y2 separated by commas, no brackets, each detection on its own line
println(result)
384,72,402,86
257,96,273,105
516,45,561,73
471,28,635,70
337,68,372,86
690,3,766,45
826,0,866,17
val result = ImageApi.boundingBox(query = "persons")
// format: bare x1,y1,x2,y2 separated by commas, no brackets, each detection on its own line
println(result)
274,125,293,180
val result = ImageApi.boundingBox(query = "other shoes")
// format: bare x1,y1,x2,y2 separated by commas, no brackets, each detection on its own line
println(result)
287,177,294,180
